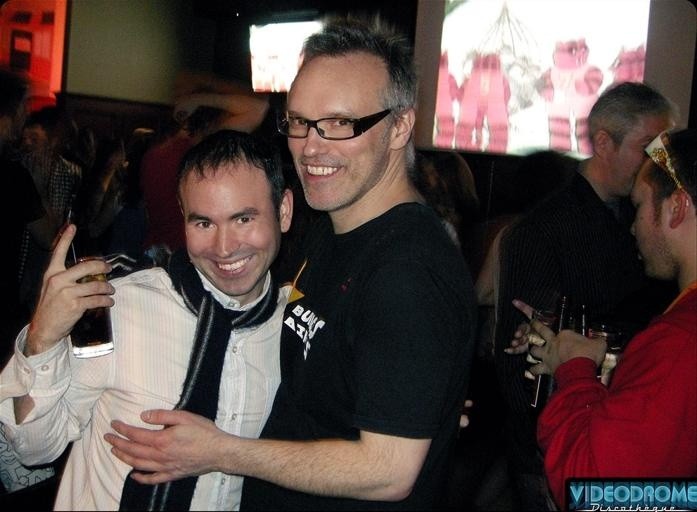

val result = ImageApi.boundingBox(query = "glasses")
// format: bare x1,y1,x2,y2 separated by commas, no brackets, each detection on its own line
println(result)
275,105,395,141
642,128,689,199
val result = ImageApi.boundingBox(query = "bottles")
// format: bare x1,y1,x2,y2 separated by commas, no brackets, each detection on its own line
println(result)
523,294,588,409
50,190,114,359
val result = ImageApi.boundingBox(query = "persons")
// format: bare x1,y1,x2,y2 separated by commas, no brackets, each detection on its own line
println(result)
101,13,473,511
0,62,696,510
